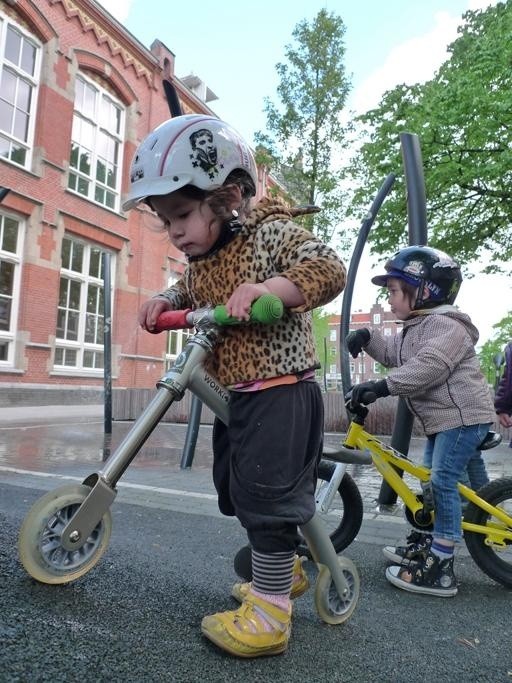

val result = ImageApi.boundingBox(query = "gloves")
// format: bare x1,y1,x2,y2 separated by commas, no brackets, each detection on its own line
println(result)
345,326,369,359
349,379,388,410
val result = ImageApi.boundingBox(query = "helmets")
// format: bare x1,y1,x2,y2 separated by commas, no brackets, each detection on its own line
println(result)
121,111,259,209
370,245,461,307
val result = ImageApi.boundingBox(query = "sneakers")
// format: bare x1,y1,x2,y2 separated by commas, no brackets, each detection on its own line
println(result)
382,532,431,565
386,552,458,598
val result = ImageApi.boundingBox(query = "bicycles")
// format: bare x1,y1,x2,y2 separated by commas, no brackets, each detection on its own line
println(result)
293,397,512,590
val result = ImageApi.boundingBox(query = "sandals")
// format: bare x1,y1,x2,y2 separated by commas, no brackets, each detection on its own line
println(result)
229,556,310,604
201,595,292,657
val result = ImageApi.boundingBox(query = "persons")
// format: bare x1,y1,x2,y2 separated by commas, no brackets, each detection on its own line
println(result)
493,341,512,450
117,111,348,660
344,244,495,597
